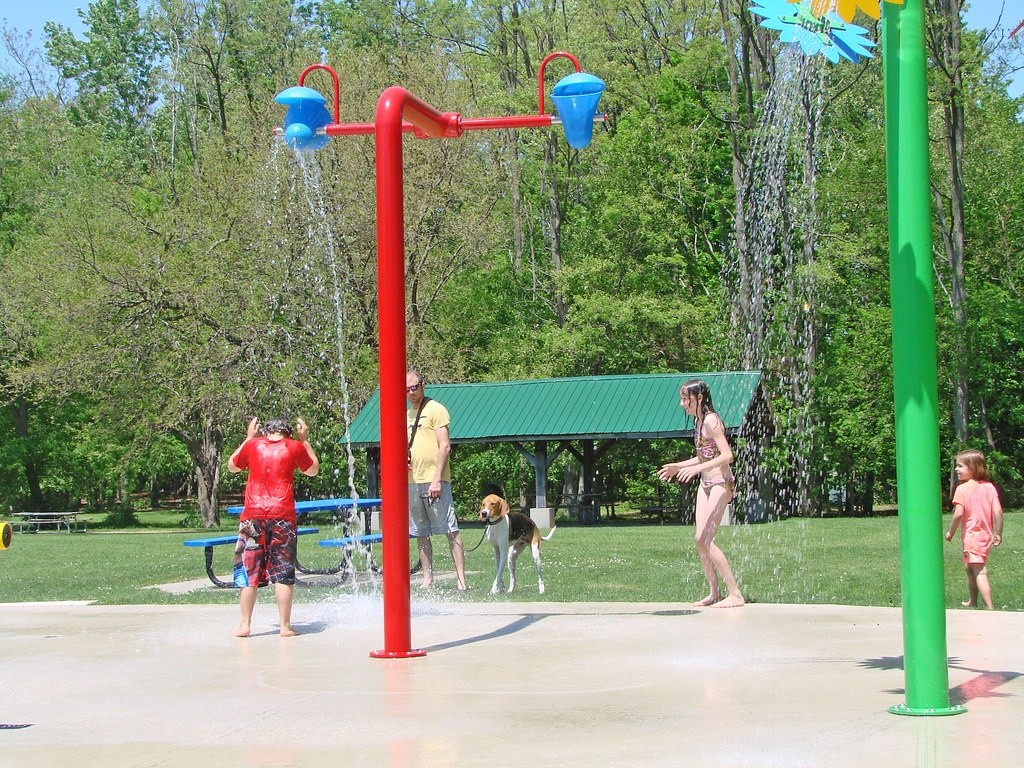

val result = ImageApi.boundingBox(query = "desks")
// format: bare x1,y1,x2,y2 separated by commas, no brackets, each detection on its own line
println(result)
183,498,425,589
549,493,602,503
10,511,88,534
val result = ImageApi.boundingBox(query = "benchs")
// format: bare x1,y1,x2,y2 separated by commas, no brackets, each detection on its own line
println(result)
183,528,319,589
640,504,687,527
564,504,620,519
547,503,600,525
17,518,71,534
318,534,422,588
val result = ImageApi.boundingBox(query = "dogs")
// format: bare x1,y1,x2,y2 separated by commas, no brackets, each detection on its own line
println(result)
479,494,557,595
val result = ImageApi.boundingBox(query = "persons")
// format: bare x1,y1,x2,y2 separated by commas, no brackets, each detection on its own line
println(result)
401,369,471,592
227,412,320,637
945,448,1003,610
657,379,745,607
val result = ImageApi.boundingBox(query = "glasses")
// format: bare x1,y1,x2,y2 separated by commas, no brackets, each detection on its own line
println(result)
406,382,421,393
